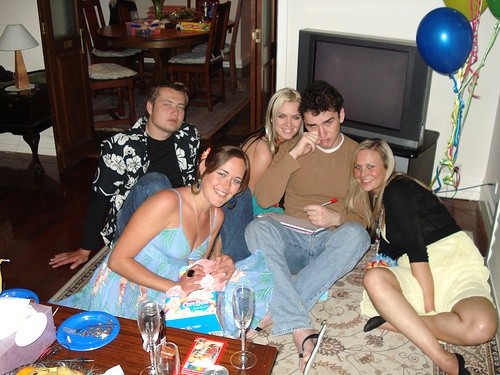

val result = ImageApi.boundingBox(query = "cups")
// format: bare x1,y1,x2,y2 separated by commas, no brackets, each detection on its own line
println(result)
154,342,180,375
139,297,166,351
203,365,229,375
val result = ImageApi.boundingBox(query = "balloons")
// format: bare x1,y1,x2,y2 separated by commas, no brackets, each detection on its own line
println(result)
416,7,474,76
486,0,500,20
443,0,488,23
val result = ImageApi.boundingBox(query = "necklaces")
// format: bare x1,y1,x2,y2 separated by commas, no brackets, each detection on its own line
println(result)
191,190,212,260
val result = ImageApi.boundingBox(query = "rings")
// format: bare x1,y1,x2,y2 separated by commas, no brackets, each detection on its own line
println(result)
187,270,195,277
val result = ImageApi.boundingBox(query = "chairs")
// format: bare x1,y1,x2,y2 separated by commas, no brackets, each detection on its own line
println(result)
79,0,242,129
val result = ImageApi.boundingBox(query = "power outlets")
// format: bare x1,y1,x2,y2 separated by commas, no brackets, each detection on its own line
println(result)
493,177,499,195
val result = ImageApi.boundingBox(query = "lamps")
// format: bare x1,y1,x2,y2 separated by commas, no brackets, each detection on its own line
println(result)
0,24,39,95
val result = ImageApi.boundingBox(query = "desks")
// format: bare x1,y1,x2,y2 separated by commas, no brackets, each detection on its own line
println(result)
6,301,279,375
0,82,54,183
96,17,218,96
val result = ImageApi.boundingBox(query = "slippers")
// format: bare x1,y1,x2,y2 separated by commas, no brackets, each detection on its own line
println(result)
239,327,263,340
297,323,326,374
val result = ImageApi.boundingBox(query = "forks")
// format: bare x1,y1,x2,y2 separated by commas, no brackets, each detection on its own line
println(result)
63,324,115,335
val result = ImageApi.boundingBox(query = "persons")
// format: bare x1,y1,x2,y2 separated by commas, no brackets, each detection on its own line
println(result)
59,145,251,339
240,88,303,214
351,140,498,375
49,82,254,272
244,81,372,375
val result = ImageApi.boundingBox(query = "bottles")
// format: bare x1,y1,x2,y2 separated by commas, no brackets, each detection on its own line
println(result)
201,0,216,19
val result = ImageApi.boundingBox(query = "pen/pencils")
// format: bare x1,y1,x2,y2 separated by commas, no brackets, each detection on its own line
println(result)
321,198,338,206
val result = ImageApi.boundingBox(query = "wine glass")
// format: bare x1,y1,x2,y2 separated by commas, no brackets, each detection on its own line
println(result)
230,285,257,370
136,298,164,375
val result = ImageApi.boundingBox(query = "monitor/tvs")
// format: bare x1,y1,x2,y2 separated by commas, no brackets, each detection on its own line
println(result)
297,28,433,151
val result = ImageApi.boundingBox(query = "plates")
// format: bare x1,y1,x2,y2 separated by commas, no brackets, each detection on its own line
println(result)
56,311,121,351
0,288,39,304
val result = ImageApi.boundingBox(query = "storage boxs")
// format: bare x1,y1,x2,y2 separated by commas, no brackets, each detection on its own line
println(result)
124,20,161,39
180,21,211,32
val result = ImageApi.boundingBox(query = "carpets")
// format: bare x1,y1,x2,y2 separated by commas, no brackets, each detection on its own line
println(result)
47,243,500,375
88,62,251,139
